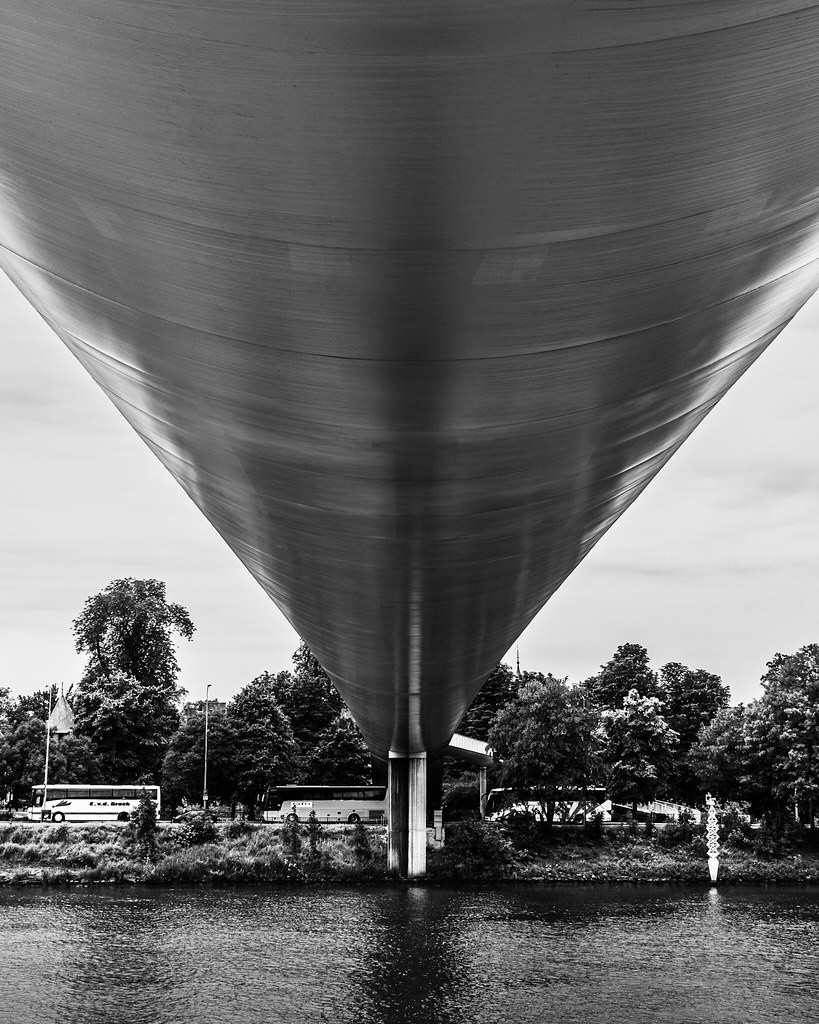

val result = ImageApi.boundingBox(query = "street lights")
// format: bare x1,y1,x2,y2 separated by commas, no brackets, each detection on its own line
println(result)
203,682,212,809
39,684,52,824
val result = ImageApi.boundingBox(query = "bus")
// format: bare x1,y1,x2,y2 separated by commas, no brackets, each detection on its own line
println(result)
256,785,390,825
26,784,161,824
480,787,612,825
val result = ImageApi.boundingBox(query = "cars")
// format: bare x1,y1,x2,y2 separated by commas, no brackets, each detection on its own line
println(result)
174,810,217,824
